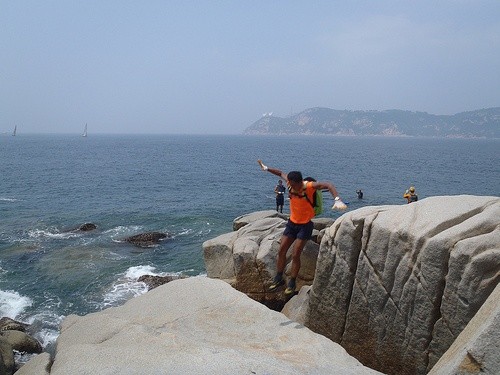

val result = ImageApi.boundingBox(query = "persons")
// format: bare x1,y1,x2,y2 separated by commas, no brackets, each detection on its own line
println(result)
257,159,347,295
404,186,418,204
274,180,286,214
356,189,363,199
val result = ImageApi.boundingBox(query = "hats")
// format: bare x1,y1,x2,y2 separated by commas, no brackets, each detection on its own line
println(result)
410,187,414,191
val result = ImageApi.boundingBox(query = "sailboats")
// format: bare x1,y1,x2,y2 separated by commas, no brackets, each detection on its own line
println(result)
82,122,88,137
12,125,16,136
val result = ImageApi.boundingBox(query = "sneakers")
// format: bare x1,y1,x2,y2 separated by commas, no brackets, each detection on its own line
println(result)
269,279,286,291
285,286,297,295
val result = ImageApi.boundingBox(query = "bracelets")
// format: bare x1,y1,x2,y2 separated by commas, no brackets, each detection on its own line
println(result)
334,196,340,201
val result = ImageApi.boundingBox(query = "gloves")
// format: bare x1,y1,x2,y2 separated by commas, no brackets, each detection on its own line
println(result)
258,160,267,171
406,190,408,193
331,199,347,209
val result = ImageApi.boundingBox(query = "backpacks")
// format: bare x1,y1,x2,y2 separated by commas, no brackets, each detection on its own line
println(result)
288,177,322,216
408,193,417,202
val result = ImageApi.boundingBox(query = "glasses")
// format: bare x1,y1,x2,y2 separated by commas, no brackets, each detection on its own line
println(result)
290,182,298,186
